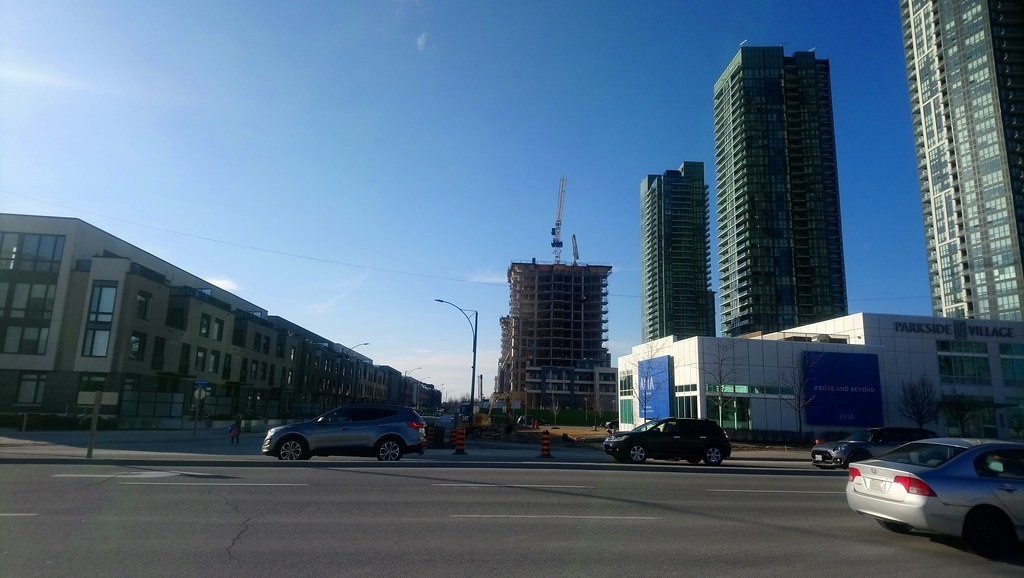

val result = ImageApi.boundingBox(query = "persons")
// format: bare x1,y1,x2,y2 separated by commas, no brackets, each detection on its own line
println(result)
228,415,242,447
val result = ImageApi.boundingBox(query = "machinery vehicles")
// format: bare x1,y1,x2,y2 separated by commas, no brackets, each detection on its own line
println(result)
454,393,509,434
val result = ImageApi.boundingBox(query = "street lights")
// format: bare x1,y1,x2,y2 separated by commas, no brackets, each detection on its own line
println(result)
612,399,616,412
416,376,430,410
404,367,422,405
523,387,527,424
435,299,478,440
330,343,368,413
584,398,589,426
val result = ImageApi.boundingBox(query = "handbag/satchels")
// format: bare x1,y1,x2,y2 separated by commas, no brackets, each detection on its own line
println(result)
232,425,238,436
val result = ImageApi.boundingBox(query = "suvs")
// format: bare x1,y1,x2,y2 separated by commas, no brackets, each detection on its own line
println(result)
261,402,428,461
601,417,732,466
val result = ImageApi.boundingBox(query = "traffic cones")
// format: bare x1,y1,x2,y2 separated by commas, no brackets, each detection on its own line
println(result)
815,436,822,445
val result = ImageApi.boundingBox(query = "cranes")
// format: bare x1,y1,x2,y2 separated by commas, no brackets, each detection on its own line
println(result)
549,179,567,266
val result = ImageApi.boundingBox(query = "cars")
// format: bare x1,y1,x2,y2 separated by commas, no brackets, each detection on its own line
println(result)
845,438,1023,555
517,415,545,426
605,419,619,429
810,427,940,470
